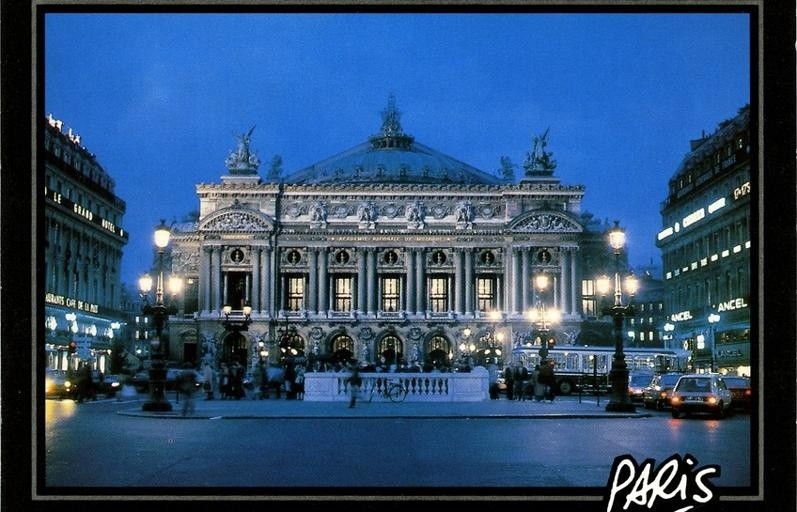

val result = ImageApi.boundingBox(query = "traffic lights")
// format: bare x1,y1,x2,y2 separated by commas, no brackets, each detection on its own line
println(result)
70,341,77,354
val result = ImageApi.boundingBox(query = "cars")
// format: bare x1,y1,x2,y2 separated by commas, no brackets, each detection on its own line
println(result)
628,373,751,419
132,369,259,393
46,368,121,400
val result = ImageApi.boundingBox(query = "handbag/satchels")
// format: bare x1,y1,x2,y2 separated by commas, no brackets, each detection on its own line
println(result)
204,381,211,390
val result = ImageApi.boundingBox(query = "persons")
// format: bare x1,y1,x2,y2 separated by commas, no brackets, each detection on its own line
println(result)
203,332,558,408
76,361,98,403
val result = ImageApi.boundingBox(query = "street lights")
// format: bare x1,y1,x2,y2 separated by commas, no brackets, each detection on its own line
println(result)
526,273,561,362
221,305,253,355
595,221,639,412
139,219,183,411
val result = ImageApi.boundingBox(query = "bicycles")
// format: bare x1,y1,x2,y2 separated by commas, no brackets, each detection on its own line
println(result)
369,376,407,403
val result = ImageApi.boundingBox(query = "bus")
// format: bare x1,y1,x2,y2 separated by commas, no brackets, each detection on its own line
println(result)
512,345,692,396
